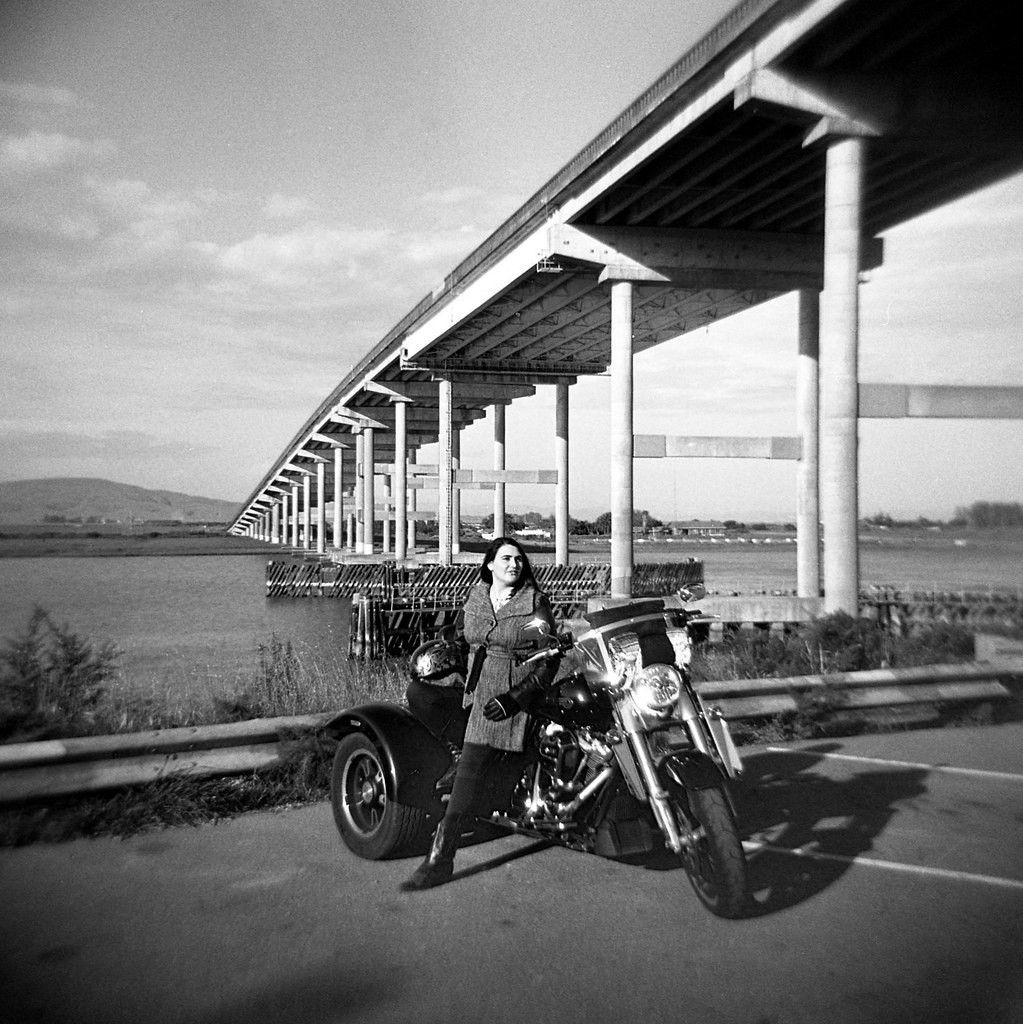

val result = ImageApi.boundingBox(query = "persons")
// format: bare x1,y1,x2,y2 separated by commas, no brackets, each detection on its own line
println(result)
398,536,561,891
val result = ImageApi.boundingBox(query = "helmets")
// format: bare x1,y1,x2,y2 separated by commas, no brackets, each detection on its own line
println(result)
414,642,459,678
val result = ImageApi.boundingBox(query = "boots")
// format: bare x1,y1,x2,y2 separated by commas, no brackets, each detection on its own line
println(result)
397,822,462,892
435,741,465,790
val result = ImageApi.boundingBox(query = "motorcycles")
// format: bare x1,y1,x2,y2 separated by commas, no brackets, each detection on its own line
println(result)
320,581,758,919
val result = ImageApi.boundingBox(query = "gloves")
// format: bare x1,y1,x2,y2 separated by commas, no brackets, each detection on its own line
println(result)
484,677,542,721
